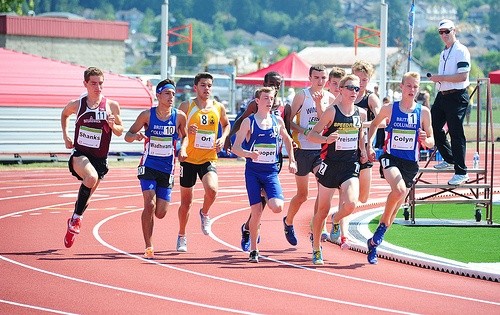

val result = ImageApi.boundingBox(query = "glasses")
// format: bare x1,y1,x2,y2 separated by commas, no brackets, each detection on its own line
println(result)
438,30,452,35
341,85,361,92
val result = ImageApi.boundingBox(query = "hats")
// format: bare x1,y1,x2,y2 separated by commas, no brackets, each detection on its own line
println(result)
438,20,454,29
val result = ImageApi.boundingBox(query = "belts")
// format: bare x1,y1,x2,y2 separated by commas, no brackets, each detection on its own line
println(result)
441,88,468,95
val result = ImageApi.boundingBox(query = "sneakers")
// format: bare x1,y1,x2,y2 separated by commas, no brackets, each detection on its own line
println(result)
340,237,350,251
241,223,251,251
257,226,260,244
249,251,259,263
64,218,75,248
434,161,454,169
312,245,324,265
309,232,328,243
367,238,378,264
200,208,211,235
282,216,297,246
69,213,83,234
373,222,388,245
330,213,341,242
448,173,470,185
144,245,154,259
176,233,187,252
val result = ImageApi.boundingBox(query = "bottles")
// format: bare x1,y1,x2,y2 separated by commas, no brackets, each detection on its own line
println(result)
473,152,480,169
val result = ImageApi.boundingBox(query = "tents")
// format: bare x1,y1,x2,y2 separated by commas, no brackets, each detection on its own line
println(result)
231,51,330,124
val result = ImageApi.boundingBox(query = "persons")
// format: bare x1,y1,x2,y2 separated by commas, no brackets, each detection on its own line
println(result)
368,72,436,264
61,67,122,248
177,72,232,253
125,78,189,260
428,19,472,184
230,87,298,263
283,60,384,265
224,72,298,252
417,86,432,108
